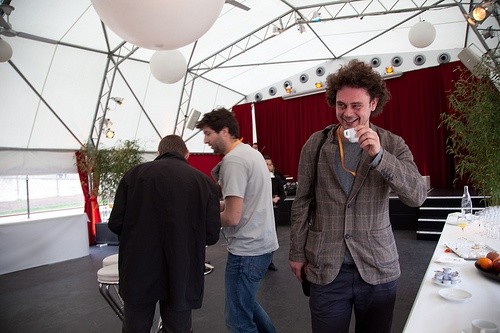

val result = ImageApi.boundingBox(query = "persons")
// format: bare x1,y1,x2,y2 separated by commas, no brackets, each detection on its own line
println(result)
211,155,286,272
108,135,221,333
196,107,280,333
288,60,427,333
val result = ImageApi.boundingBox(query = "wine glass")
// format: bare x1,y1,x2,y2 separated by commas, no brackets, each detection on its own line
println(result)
457,215,468,240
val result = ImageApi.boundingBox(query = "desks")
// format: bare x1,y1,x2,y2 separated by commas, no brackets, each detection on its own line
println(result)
401,212,500,333
96,253,162,333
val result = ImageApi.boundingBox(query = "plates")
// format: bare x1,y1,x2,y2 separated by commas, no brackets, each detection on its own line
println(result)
431,273,464,287
438,287,473,302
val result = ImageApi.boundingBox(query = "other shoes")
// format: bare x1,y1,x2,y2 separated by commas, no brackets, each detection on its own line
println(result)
269,263,277,270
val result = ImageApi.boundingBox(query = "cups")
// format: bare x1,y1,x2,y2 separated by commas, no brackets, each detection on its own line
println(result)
472,319,498,333
343,128,359,143
474,204,500,240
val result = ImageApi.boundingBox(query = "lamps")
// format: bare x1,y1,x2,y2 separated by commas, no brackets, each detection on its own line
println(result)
457,43,491,78
185,107,201,130
467,1,497,25
100,97,124,138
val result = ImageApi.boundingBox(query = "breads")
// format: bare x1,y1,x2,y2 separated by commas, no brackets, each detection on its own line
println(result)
476,252,500,271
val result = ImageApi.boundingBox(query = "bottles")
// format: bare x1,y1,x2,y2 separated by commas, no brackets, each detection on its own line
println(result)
461,186,472,219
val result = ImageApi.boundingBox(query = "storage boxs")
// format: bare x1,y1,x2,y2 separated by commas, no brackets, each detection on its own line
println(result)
422,176,431,190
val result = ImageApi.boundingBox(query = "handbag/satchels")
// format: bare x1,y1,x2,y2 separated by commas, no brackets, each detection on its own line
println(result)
301,265,311,296
